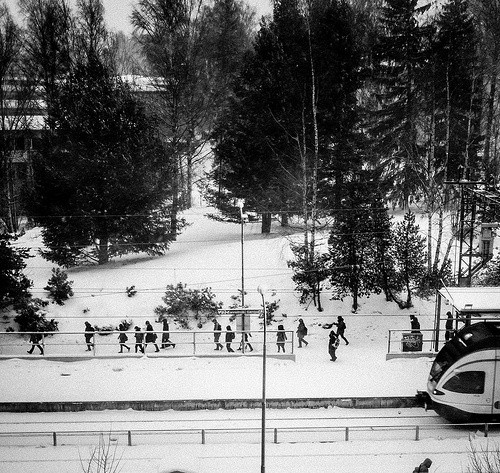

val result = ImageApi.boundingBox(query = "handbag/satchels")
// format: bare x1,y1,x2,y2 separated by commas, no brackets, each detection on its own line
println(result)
302,328,307,335
153,334,158,338
332,335,340,349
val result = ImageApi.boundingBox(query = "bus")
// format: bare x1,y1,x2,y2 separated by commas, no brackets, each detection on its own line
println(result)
414,320,500,430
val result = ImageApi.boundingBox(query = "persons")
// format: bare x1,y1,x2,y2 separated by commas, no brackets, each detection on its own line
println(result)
296,319,309,348
27,322,43,355
328,330,340,361
118,325,130,353
333,316,349,345
212,319,223,350
84,321,94,351
134,326,144,353
444,311,454,344
410,315,420,333
237,333,253,352
140,320,160,353
226,325,234,352
160,319,176,349
276,325,287,353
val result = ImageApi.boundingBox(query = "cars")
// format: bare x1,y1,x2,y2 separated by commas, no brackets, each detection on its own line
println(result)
240,211,260,223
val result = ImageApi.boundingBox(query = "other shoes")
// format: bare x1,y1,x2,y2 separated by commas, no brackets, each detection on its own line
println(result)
330,357,337,362
346,342,349,345
118,344,175,353
86,349,91,351
214,346,253,352
27,351,32,355
298,343,308,348
40,352,43,355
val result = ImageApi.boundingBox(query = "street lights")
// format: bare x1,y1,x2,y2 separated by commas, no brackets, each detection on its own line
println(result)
241,214,249,354
257,287,267,473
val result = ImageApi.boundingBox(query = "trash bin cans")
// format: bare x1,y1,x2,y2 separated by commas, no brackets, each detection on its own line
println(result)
402,331,423,352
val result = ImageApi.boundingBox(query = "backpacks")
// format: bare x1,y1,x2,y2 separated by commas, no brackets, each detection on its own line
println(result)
413,463,425,473
232,333,235,339
